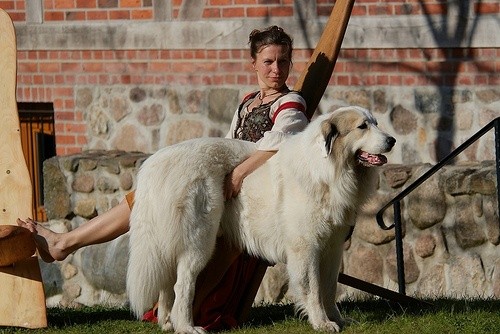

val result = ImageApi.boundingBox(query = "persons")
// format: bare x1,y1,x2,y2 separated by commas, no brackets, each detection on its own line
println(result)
17,26,306,332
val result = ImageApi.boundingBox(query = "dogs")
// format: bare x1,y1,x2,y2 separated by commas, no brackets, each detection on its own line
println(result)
122,104,397,334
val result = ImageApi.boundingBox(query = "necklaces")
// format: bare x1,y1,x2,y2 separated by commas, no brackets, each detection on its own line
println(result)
258,92,281,106
